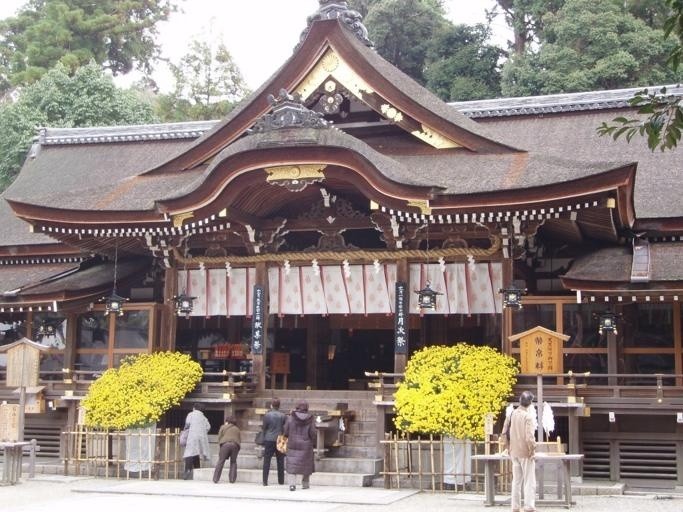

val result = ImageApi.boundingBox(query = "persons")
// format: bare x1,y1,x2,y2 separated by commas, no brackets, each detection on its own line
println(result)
183,402,211,481
508,390,538,512
262,398,287,486
213,417,241,483
283,400,317,491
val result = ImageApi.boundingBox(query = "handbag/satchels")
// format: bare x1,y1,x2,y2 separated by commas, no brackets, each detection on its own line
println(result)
180,430,187,447
276,435,288,455
506,410,516,441
255,432,265,445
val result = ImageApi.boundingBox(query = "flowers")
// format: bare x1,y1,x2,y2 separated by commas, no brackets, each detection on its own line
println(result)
393,344,520,439
79,350,204,427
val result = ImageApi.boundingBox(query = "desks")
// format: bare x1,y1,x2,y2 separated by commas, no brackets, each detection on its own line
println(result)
471,453,581,509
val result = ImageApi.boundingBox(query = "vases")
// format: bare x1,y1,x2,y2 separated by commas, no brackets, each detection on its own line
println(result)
126,422,155,476
443,433,471,486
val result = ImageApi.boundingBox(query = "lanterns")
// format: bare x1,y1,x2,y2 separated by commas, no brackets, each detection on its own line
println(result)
167,295,198,319
98,295,130,317
37,321,57,335
591,311,623,335
414,288,444,307
497,286,528,309
